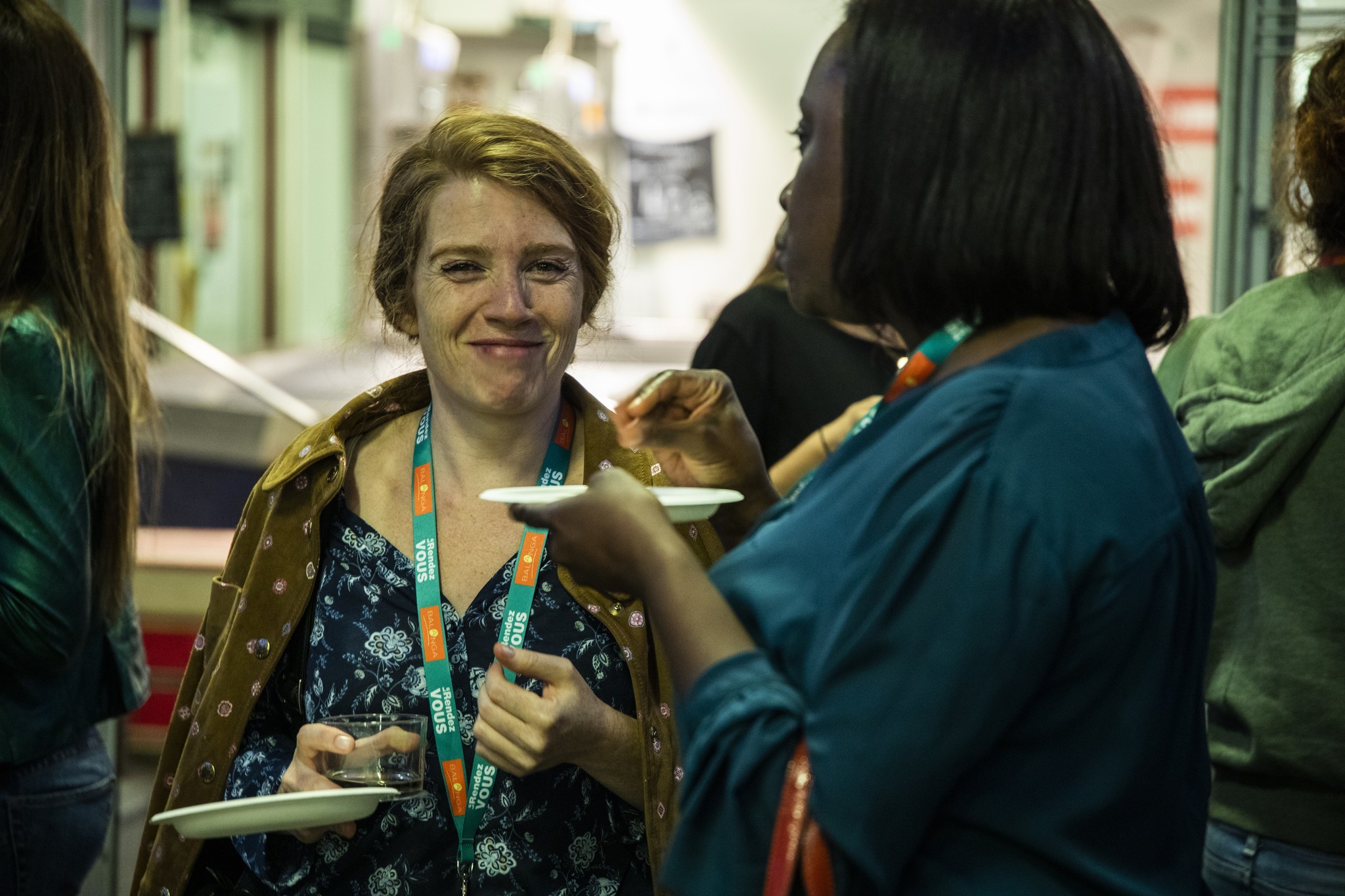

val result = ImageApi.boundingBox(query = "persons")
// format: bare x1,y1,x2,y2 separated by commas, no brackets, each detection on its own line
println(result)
199,107,736,896
691,264,906,501
0,0,150,896
1151,32,1345,895
504,4,1228,896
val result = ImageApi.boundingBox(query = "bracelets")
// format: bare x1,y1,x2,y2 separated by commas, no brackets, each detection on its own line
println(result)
818,425,831,457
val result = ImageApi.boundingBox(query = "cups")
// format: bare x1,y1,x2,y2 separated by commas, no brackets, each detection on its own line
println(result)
319,714,427,802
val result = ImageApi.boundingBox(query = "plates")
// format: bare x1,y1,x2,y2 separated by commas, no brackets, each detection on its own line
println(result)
476,485,745,526
150,785,402,841
